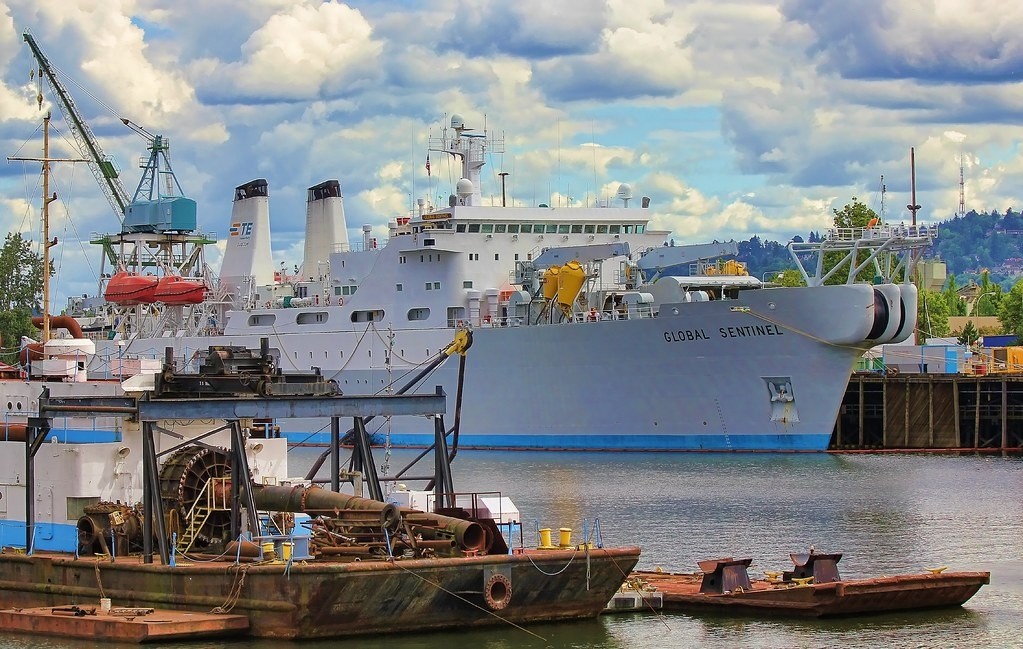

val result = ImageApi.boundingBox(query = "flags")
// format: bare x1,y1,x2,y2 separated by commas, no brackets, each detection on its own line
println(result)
426,154,431,175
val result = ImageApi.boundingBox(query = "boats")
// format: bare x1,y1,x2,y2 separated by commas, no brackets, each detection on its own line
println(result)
152,274,209,306
19,29,921,457
603,546,992,612
104,271,161,306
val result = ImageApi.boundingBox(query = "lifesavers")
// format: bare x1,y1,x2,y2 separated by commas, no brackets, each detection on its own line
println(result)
338,298,343,306
266,302,271,308
327,299,332,305
458,320,463,326
